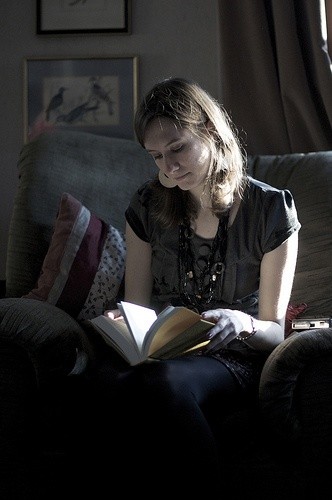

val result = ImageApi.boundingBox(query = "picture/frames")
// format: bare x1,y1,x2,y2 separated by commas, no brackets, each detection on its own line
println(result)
36,0,132,33
22,53,140,143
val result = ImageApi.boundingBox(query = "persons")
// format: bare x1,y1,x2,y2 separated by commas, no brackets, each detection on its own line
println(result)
82,77,301,500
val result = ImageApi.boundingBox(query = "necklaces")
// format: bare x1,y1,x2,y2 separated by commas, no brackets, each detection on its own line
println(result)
179,208,229,316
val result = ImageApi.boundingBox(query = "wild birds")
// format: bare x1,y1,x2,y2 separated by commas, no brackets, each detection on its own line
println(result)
44,87,68,122
87,80,116,116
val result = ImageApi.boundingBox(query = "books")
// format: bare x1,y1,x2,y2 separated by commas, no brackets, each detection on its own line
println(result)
87,301,216,367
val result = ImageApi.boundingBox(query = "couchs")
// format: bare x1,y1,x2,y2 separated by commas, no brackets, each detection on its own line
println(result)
3,126,331,495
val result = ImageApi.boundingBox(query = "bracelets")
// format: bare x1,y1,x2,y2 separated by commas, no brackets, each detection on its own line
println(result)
235,316,257,340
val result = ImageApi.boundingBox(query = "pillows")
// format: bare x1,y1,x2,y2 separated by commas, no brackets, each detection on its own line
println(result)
23,192,130,330
